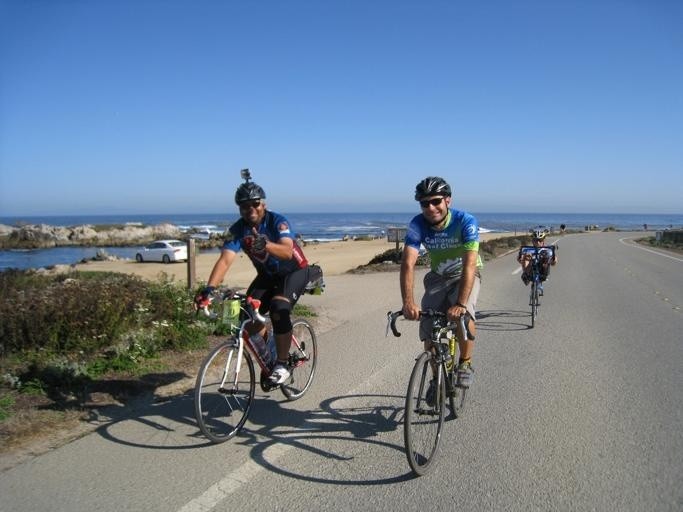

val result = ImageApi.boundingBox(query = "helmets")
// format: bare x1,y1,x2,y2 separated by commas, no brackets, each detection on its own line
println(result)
416,177,451,201
532,230,545,240
235,182,265,204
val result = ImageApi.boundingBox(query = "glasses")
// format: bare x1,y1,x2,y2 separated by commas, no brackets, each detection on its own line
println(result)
534,239,542,242
420,198,444,208
241,202,260,209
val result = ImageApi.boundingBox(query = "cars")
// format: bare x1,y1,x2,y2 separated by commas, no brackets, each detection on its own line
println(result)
135,239,187,263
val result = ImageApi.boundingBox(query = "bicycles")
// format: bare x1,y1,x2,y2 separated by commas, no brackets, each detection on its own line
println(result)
193,286,317,445
384,308,468,477
526,249,550,328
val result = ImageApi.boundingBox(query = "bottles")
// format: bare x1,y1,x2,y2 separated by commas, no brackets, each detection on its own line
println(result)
251,332,271,364
441,342,453,373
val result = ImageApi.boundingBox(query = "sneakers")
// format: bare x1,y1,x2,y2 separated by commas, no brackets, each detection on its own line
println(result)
268,365,291,386
426,379,437,407
457,363,474,388
522,273,530,285
540,267,547,281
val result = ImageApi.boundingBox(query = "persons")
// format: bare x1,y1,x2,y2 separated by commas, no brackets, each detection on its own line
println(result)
193,182,309,385
517,230,557,286
399,176,484,408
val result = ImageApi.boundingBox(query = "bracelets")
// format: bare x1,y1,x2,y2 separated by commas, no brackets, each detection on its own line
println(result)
456,302,467,308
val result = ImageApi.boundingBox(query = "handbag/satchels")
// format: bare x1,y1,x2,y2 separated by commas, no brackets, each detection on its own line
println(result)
306,265,324,293
